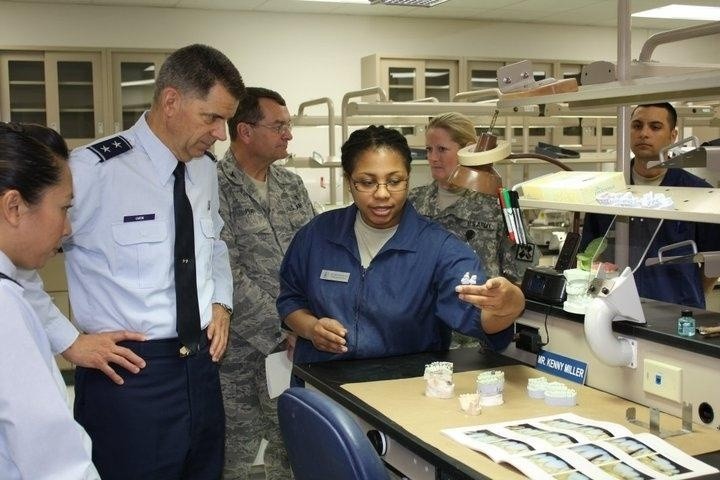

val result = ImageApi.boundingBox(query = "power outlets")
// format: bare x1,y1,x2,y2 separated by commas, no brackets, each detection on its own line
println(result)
515,321,540,358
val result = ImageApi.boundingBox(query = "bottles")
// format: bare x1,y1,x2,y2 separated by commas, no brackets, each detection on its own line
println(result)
678,310,696,336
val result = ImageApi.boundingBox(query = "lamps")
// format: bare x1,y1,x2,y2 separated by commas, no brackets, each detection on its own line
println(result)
447,112,583,305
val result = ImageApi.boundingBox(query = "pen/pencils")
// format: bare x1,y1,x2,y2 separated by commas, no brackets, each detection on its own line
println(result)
497,186,528,245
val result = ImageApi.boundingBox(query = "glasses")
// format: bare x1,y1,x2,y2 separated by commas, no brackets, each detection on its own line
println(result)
347,173,411,192
246,121,293,134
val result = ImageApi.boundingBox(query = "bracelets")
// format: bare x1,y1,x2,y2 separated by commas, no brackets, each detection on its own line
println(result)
215,302,233,314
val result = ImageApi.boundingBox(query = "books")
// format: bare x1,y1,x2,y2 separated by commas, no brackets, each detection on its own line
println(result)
443,411,719,480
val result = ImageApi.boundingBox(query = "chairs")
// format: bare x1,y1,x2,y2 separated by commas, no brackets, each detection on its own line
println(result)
278,388,390,480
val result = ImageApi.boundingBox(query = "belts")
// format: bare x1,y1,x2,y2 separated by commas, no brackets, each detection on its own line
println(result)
120,332,206,359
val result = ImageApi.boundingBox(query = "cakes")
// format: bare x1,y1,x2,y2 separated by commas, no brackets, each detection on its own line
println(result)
423,360,455,399
544,380,577,407
476,368,504,407
527,377,547,399
458,392,481,414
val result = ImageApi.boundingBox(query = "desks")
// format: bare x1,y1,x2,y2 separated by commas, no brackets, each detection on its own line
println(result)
293,346,720,480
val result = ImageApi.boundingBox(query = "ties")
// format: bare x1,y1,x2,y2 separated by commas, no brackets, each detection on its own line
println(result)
172,161,202,347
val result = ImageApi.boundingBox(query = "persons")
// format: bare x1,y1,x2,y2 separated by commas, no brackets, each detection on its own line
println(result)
18,46,242,479
276,124,525,390
208,86,317,478
578,103,706,309
405,112,520,349
0,123,103,480
